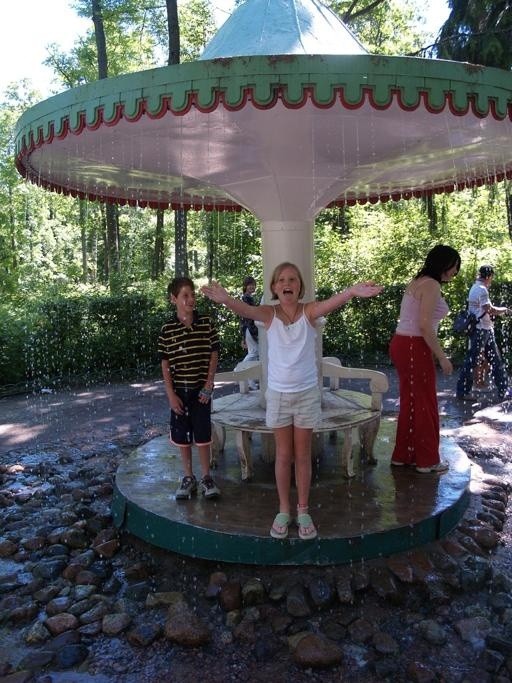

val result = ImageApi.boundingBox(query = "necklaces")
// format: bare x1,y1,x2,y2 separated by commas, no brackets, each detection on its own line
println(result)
281,303,299,325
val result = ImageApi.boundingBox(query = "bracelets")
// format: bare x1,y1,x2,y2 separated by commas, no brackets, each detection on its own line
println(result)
202,386,212,400
343,288,351,300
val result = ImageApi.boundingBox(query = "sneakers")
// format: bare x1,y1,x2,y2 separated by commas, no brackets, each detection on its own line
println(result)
175,474,197,499
391,461,416,466
415,459,450,473
457,393,476,400
198,474,220,498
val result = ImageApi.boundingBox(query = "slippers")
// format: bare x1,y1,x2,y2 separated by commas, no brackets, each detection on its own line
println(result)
295,514,317,540
270,512,292,538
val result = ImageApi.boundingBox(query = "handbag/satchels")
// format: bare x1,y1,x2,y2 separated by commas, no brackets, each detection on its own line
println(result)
452,310,478,337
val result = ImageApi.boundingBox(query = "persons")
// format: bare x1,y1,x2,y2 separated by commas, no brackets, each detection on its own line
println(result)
389,245,461,473
201,262,385,541
234,275,259,388
457,263,512,399
157,275,223,500
